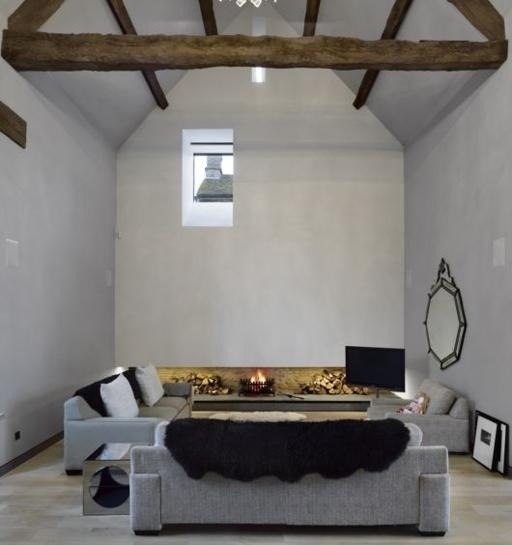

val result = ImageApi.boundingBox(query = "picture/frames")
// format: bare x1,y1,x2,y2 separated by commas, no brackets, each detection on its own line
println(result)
468,410,508,475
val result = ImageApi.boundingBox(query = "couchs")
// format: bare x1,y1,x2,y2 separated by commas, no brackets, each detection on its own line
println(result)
128,419,450,534
366,380,469,453
61,365,193,475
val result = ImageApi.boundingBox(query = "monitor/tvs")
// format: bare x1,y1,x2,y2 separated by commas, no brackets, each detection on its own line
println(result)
345,345,406,392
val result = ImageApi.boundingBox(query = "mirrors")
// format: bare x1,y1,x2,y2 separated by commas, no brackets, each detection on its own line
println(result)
418,258,468,369
